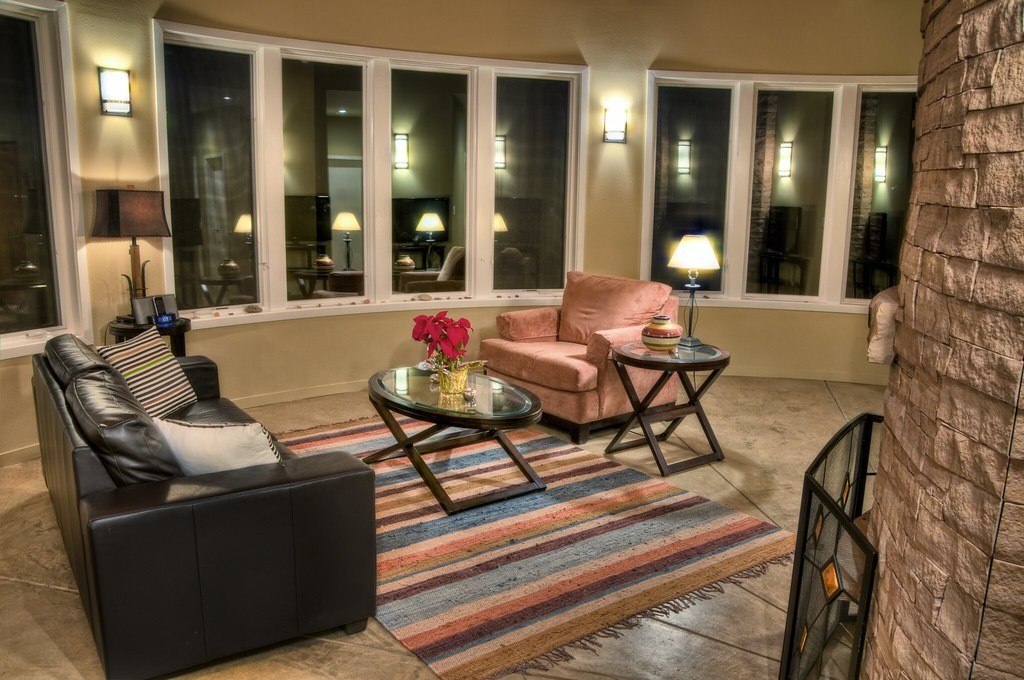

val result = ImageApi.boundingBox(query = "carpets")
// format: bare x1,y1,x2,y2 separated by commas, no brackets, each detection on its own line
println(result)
277,408,801,680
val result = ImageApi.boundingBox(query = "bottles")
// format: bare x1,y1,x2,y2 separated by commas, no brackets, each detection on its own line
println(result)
641,315,682,351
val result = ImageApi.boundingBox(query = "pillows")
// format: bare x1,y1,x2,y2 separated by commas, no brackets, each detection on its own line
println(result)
153,414,284,485
95,323,197,419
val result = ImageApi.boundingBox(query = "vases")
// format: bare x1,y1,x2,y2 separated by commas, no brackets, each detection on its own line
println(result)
426,351,460,377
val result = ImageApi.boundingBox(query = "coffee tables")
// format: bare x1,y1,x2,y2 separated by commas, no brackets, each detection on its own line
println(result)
361,363,544,516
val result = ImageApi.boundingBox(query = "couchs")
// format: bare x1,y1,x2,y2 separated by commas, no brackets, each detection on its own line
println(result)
398,246,471,293
30,335,378,680
231,274,257,304
312,268,362,298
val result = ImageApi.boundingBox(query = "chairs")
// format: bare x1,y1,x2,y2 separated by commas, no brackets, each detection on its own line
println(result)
473,271,677,446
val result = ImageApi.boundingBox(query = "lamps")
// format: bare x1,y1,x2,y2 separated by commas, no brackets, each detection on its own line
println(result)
678,140,691,173
395,132,411,169
495,135,506,168
332,211,362,270
668,234,715,347
493,212,508,235
877,146,889,183
600,107,628,143
92,185,171,322
99,63,132,116
413,213,448,270
778,142,792,176
234,213,252,272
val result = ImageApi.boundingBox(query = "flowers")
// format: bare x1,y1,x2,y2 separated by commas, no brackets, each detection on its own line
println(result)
410,313,471,362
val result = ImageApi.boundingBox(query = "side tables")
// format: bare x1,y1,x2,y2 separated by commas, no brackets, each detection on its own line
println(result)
110,316,194,358
599,344,730,477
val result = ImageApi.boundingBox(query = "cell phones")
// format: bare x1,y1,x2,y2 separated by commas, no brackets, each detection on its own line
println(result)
152,297,166,316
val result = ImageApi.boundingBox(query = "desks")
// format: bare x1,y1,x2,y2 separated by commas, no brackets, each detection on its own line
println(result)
291,271,336,297
198,278,253,307
